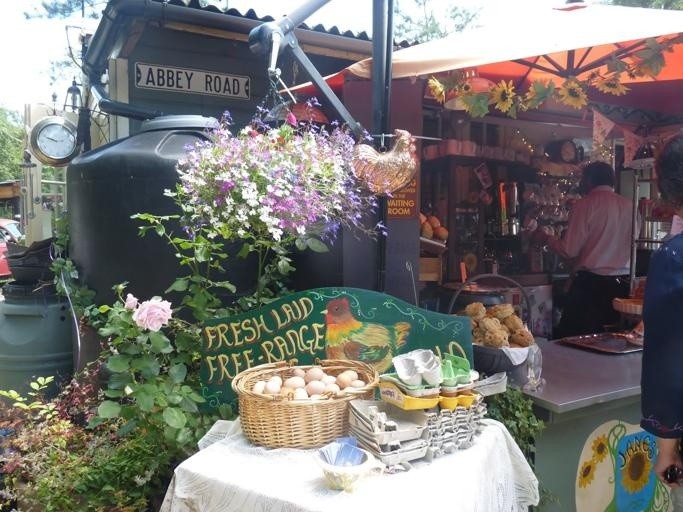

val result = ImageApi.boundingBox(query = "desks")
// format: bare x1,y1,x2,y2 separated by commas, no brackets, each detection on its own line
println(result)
486,330,680,512
167,407,538,512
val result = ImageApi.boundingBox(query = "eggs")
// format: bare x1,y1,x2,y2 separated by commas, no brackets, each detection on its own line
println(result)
254,368,364,399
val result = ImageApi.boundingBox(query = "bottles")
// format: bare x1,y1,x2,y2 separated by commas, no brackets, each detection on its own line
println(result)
497,180,521,237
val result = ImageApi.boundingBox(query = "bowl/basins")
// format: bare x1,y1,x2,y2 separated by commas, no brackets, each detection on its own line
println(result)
7,248,56,283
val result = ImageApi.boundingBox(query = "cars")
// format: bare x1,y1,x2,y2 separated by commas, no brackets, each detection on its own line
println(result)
0,219,22,276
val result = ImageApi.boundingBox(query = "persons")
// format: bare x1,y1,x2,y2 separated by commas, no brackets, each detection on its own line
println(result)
636,135,682,511
522,159,644,341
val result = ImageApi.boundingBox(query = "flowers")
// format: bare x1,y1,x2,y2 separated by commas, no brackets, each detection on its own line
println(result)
426,32,683,118
0,94,396,512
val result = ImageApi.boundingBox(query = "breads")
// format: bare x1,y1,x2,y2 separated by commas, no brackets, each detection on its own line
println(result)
465,302,534,348
420,213,448,240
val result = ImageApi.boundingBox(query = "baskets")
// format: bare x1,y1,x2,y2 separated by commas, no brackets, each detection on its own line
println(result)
472,344,531,374
230,357,380,449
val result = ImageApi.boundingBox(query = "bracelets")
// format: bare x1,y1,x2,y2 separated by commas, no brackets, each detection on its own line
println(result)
543,234,554,246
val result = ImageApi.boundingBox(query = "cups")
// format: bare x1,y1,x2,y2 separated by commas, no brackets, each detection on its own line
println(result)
315,442,375,493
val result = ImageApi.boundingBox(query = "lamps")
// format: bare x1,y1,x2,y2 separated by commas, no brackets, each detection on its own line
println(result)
444,65,501,111
631,125,663,171
